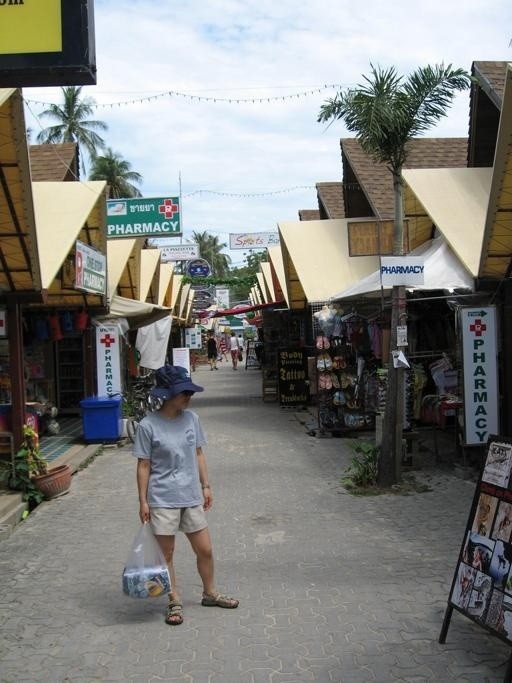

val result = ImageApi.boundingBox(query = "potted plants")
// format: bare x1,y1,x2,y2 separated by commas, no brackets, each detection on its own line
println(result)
118,391,136,437
15,424,73,519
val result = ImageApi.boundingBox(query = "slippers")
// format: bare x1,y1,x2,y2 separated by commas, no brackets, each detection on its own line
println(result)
340,356,347,369
345,392,354,408
326,374,333,390
355,394,361,408
360,415,365,426
340,372,348,389
319,395,327,410
320,375,326,389
316,335,323,350
365,415,373,425
281,351,307,402
333,356,340,369
323,336,331,349
326,394,333,410
331,372,340,389
324,353,333,370
317,354,325,372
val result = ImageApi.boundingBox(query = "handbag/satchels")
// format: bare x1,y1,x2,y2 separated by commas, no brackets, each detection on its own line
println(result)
238,353,242,361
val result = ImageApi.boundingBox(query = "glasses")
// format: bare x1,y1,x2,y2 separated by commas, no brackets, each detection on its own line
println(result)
375,371,386,413
404,367,415,429
182,390,194,396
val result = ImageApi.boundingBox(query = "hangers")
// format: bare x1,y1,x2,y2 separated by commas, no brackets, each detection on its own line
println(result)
442,350,455,371
340,306,368,321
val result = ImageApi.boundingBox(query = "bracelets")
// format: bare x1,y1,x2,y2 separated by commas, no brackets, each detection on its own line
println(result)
202,485,209,489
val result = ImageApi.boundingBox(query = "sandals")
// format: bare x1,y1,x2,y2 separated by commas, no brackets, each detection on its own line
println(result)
165,598,183,624
202,590,239,608
340,391,346,405
333,391,340,406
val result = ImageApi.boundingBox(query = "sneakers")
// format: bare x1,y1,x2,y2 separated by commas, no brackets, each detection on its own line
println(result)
319,408,359,428
331,334,353,355
211,367,213,371
214,366,218,370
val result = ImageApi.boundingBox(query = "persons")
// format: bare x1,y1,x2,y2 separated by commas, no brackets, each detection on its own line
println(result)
314,305,337,336
130,365,240,626
328,303,344,336
202,330,246,370
447,441,511,644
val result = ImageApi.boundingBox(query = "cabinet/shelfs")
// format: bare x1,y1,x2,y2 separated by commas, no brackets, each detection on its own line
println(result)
245,340,262,370
55,326,86,414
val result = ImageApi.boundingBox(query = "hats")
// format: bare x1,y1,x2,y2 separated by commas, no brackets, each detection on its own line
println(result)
149,366,204,402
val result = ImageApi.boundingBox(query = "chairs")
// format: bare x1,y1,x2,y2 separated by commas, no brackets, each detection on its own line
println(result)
262,368,279,401
0,426,15,470
